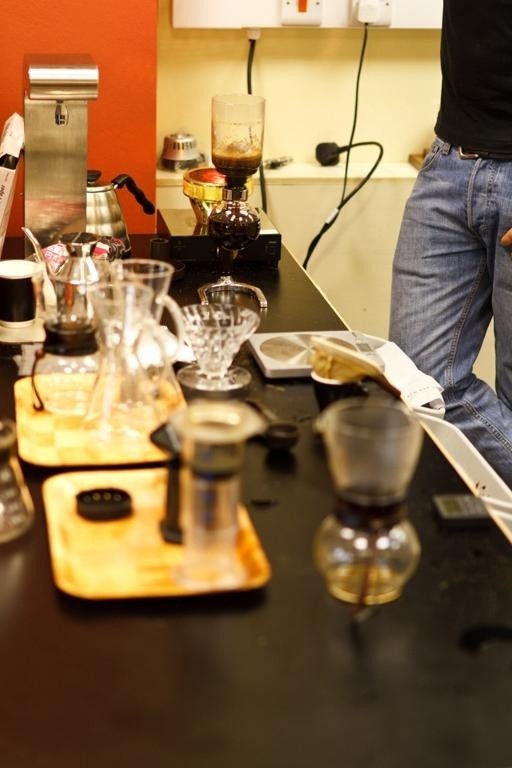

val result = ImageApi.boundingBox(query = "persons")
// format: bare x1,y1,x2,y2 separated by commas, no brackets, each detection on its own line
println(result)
389,0,512,490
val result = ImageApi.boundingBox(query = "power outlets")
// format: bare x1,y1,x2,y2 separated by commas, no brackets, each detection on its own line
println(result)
347,0,391,28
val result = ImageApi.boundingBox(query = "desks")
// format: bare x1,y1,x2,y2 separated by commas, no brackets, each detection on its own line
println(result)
0,233,512,768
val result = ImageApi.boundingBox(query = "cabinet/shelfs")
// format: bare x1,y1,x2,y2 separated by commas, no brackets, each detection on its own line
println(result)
156,158,497,393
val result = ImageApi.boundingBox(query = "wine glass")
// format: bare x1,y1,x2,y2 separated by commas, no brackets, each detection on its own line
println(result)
174,302,261,394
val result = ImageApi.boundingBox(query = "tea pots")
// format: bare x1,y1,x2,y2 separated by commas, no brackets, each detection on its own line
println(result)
19,226,126,335
84,168,157,263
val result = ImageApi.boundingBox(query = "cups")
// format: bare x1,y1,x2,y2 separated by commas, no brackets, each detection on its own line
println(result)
314,395,426,607
83,258,183,431
209,92,267,170
